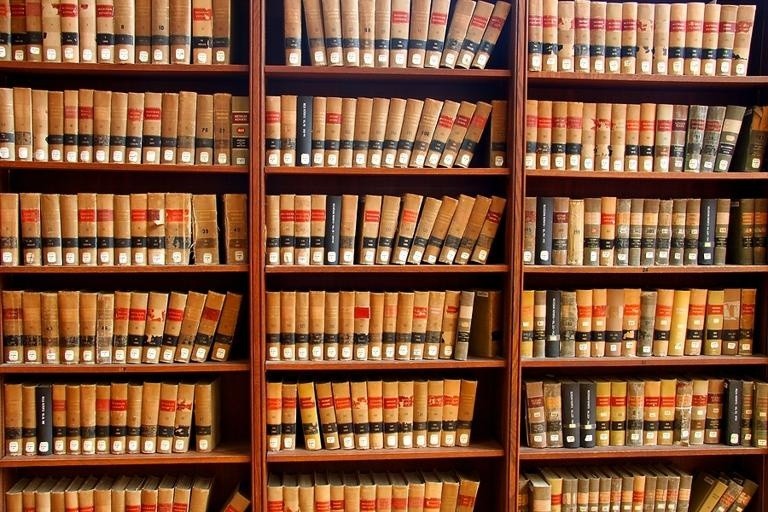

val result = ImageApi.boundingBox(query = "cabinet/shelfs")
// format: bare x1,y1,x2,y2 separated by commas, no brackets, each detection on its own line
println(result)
2,2,263,511
259,1,518,511
519,0,768,510
